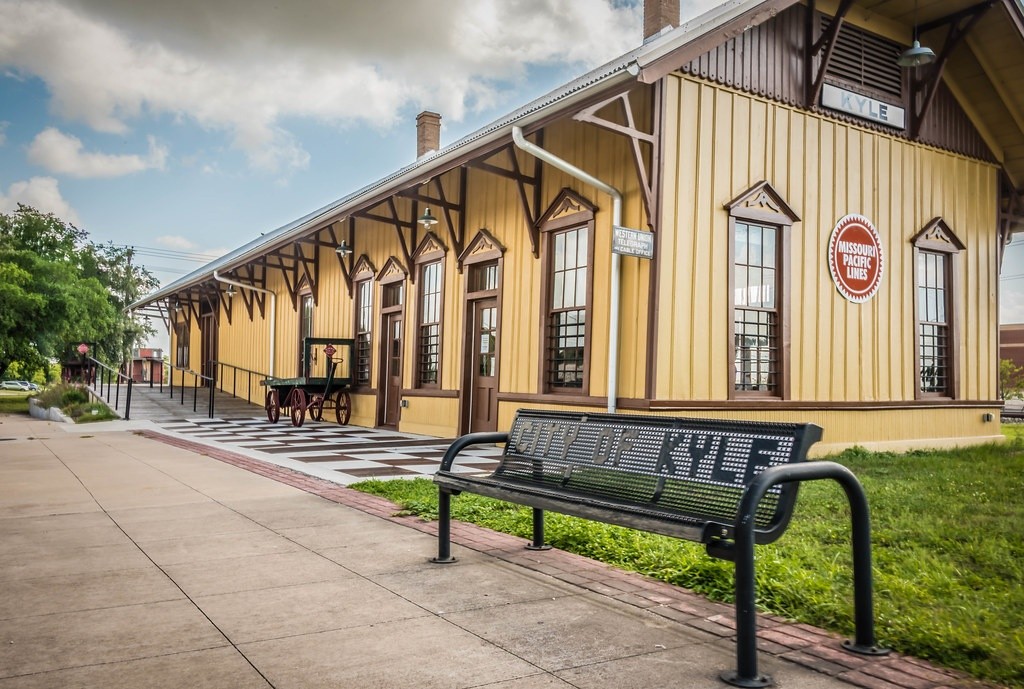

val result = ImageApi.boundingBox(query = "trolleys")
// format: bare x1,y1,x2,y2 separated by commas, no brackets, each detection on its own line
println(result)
259,336,358,429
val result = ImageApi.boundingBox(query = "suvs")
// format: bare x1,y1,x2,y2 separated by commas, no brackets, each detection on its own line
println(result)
1,381,29,391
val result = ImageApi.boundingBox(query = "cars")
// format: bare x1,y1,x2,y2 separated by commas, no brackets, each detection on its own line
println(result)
29,383,38,390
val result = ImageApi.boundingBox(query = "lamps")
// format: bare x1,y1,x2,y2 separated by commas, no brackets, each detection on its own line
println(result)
895,0,936,68
173,301,182,313
144,315,149,321
416,182,438,230
335,222,353,258
226,284,237,297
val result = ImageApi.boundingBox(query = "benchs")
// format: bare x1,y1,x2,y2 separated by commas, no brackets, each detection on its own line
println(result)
427,408,891,688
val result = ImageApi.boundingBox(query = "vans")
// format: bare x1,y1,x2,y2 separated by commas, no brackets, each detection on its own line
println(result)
18,381,31,389
